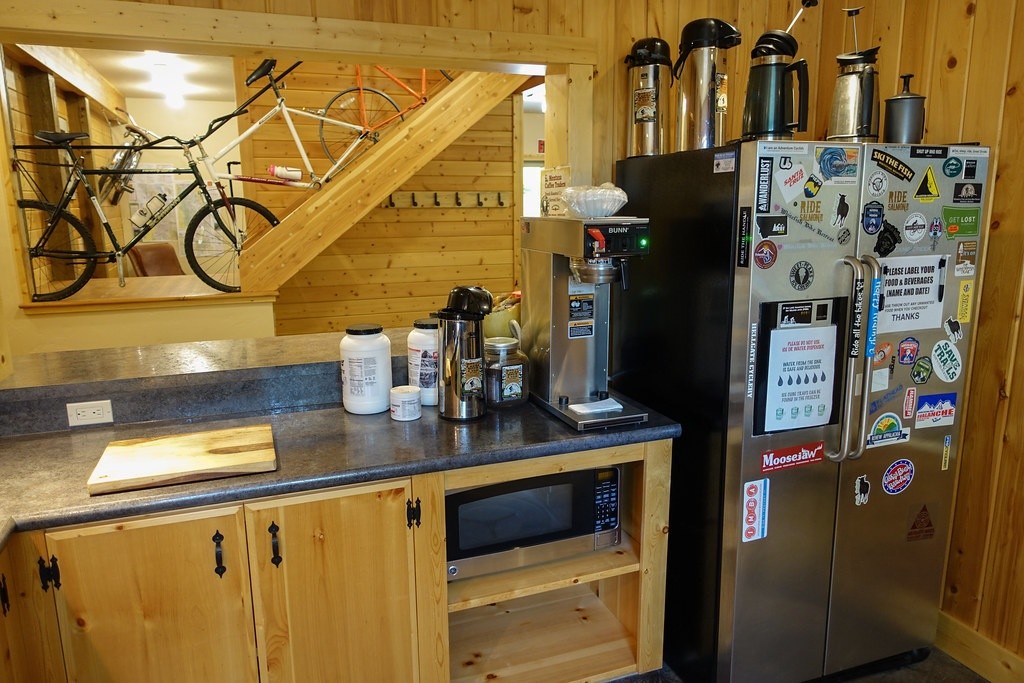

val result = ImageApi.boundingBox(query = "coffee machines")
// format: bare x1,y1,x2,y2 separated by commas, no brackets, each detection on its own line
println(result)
519,214,650,430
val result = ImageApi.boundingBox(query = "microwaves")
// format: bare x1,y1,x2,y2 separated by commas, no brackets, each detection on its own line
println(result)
448,467,628,584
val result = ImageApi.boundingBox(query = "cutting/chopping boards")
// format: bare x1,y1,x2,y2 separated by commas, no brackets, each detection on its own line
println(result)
84,420,277,497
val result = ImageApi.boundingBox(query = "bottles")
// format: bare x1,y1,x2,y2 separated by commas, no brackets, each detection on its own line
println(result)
335,318,438,420
264,164,305,180
127,193,167,228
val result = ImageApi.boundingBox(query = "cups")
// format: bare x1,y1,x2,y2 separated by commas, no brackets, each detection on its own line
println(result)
885,72,926,146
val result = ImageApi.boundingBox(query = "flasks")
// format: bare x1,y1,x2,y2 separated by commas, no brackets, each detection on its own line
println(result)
428,283,488,422
621,37,674,158
743,26,809,141
673,18,739,151
825,47,884,145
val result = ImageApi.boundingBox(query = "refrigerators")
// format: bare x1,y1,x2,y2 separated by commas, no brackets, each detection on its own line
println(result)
612,136,1005,681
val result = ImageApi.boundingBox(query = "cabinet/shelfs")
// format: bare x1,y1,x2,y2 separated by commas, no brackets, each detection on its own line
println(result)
0,439,671,683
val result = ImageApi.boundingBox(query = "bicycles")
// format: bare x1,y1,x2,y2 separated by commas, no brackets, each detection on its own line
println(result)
11,57,456,300
